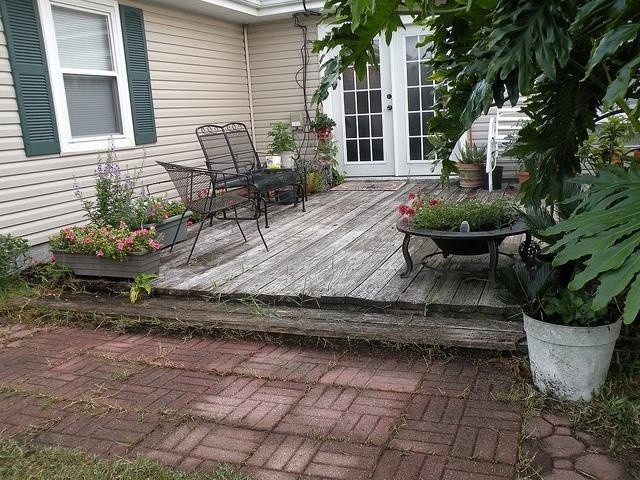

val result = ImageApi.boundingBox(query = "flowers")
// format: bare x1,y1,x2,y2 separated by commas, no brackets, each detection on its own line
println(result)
53,129,169,260
396,189,446,228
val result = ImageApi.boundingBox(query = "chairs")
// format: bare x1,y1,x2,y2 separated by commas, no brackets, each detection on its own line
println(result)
154,121,308,266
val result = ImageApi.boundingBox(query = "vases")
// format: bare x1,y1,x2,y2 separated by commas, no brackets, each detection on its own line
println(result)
396,215,538,290
50,210,194,278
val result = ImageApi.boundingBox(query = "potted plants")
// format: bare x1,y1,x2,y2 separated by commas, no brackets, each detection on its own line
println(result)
449,139,498,189
487,172,624,402
309,111,339,140
